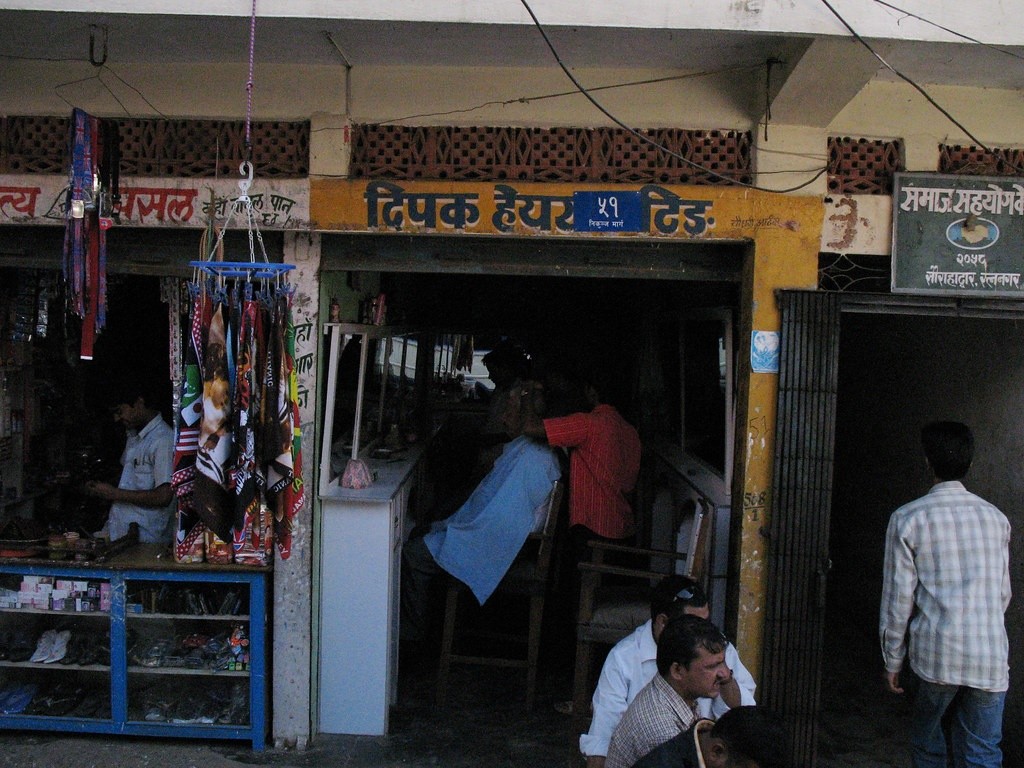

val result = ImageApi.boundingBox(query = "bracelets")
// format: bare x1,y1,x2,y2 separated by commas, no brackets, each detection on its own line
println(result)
120,489,127,502
521,391,528,395
720,670,733,684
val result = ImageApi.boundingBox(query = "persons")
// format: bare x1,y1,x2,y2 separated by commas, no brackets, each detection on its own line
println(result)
629,707,794,768
606,613,729,768
538,367,583,456
578,575,757,768
91,383,175,542
519,375,640,584
483,347,528,468
879,422,1012,768
400,391,562,660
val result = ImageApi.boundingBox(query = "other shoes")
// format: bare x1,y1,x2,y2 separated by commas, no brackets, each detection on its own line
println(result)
0,675,250,724
553,694,576,715
1,626,222,669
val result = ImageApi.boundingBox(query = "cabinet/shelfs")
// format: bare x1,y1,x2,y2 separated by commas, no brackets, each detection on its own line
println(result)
0,541,271,754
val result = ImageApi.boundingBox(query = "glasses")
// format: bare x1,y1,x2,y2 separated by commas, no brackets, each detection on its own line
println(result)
673,582,706,602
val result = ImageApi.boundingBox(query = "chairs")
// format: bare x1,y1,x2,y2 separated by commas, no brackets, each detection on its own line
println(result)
435,479,563,701
571,499,708,710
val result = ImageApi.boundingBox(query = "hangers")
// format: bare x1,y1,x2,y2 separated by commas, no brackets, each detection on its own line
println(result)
54,62,132,123
